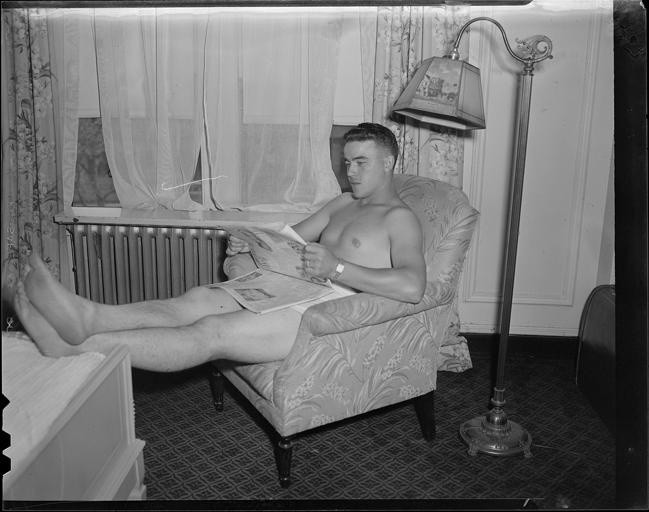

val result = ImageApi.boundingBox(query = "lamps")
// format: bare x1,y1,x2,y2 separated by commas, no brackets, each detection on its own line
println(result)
392,17,553,460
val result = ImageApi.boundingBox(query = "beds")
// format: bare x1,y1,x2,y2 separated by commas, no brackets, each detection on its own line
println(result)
0,332,147,502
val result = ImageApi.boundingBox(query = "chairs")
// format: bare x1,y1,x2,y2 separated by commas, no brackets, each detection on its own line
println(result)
212,174,480,487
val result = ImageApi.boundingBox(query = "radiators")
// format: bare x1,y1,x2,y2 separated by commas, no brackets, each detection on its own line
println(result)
55,215,287,305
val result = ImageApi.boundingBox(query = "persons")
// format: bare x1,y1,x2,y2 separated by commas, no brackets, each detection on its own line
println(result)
12,122,427,374
